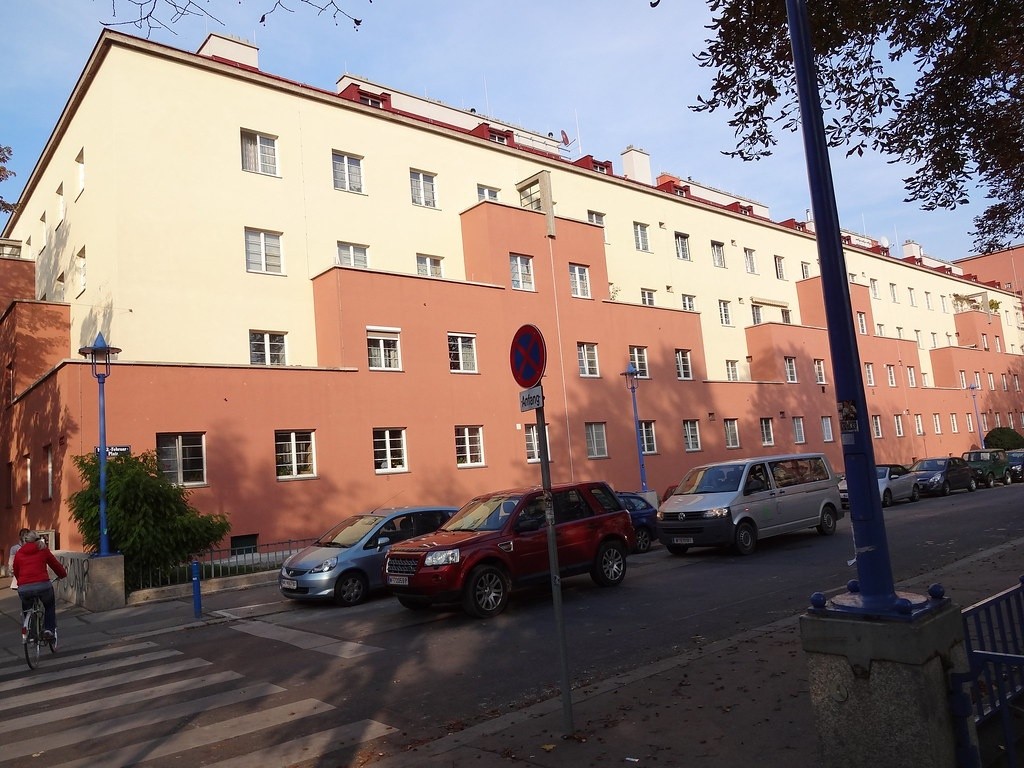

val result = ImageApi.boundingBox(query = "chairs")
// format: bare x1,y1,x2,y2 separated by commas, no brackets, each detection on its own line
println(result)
382,518,413,537
499,501,515,524
711,470,738,480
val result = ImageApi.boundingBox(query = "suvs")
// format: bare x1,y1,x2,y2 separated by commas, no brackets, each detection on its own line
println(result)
380,476,638,619
961,448,1013,488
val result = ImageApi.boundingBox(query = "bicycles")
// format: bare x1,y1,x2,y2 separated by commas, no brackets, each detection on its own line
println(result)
20,576,61,671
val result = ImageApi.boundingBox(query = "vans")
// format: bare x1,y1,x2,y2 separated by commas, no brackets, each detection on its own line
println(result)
656,452,845,557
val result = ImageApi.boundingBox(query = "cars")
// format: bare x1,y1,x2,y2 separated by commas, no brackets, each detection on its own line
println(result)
908,456,978,497
593,491,658,555
837,463,920,509
1004,448,1024,483
278,506,461,608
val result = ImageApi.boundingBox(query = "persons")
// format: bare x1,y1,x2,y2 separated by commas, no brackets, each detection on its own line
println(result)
8,528,68,643
519,498,545,531
745,470,764,490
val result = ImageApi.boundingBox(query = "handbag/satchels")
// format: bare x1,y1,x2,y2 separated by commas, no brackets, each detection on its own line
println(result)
10,576,19,590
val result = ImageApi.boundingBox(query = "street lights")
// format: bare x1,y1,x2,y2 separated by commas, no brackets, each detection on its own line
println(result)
77,331,122,557
620,362,648,492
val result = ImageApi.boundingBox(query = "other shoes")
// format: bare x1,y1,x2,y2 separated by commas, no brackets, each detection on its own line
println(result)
44,630,56,644
